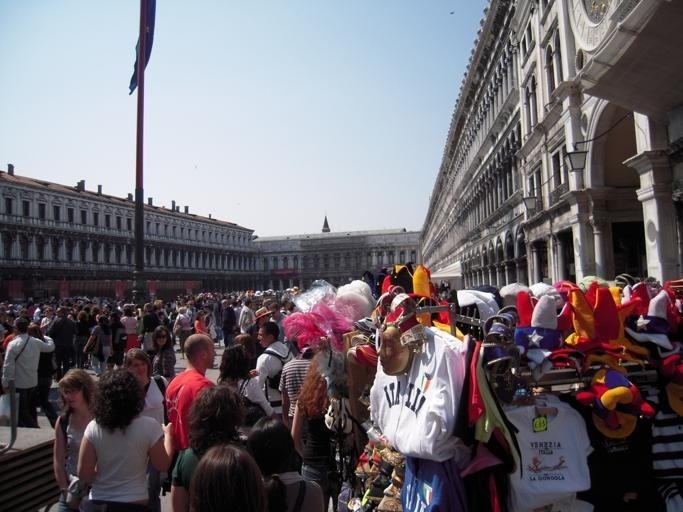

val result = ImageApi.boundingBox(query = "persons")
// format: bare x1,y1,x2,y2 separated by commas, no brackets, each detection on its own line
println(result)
0,274,683,512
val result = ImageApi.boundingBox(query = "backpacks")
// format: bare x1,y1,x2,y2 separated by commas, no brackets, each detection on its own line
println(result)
114,327,128,347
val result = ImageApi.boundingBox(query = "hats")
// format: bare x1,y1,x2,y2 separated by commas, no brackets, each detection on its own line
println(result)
11,316,28,327
255,307,273,320
380,324,414,376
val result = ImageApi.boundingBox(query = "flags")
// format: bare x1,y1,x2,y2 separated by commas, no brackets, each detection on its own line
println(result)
126,0,159,94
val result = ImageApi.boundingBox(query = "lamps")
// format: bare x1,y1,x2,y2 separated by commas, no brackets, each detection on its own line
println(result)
565,108,635,173
523,161,566,210
487,220,506,235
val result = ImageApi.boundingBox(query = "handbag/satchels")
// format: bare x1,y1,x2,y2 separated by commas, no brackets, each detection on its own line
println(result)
174,324,183,338
90,340,104,363
